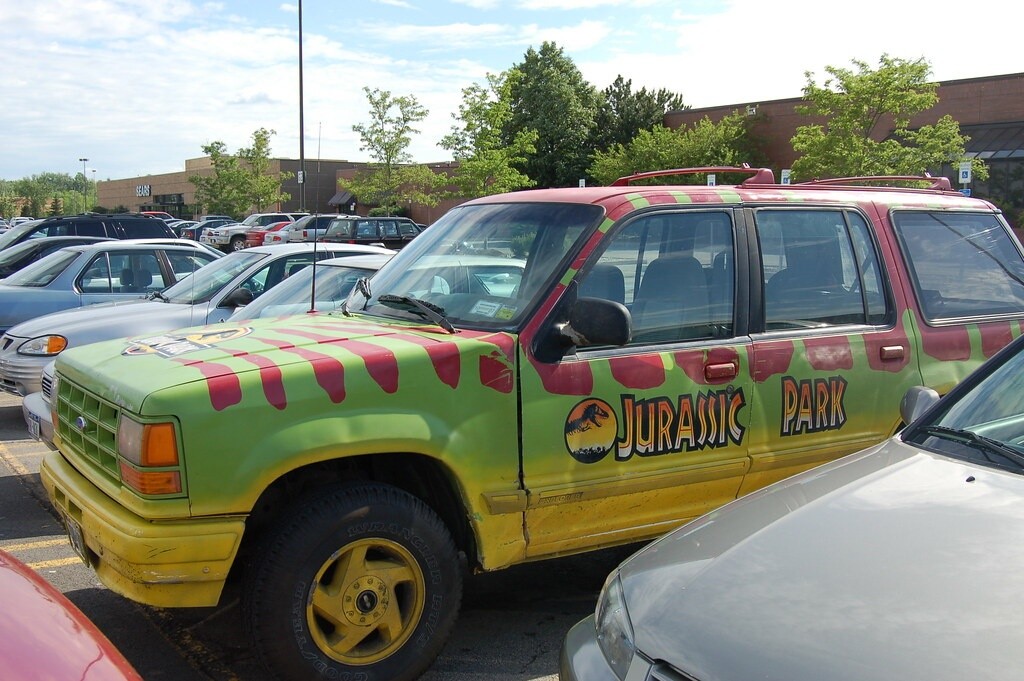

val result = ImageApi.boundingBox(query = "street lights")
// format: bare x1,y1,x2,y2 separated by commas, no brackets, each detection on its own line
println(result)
79,158,89,211
93,170,97,206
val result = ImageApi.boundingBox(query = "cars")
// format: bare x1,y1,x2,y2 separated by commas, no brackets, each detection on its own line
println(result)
559,334,1024,681
0,217,48,237
141,212,242,249
0,240,400,394
357,221,428,234
246,222,292,247
20,257,533,447
0,234,264,333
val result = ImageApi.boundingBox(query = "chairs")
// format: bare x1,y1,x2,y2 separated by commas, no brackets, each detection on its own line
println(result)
578,263,625,306
630,255,710,343
132,269,154,293
120,269,134,292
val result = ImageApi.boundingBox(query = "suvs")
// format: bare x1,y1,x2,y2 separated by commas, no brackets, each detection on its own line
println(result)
322,216,453,254
287,215,360,241
213,213,311,253
40,188,1024,681
0,214,180,245
